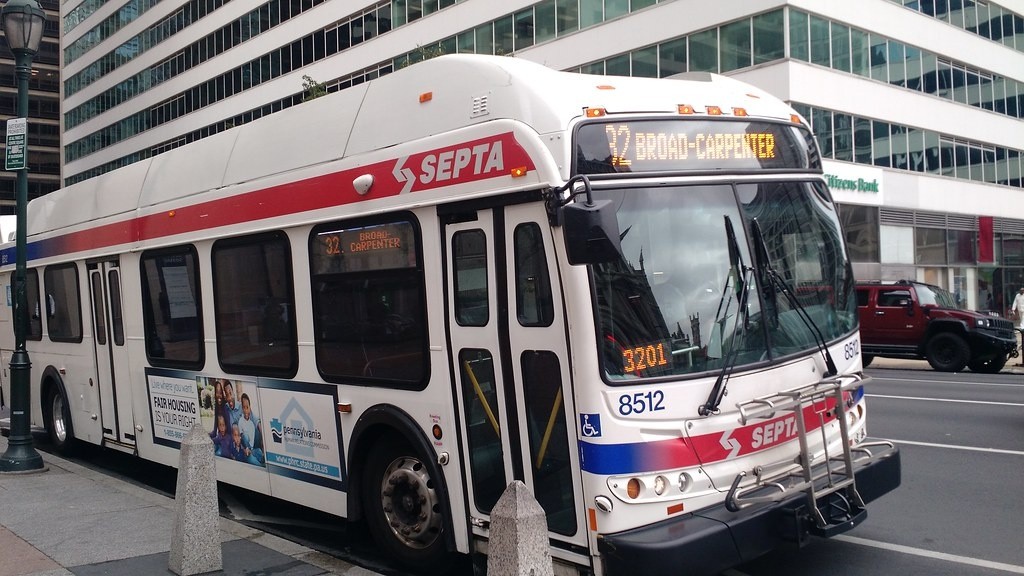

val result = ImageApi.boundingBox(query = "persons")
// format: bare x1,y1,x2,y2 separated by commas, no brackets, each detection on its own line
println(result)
208,380,264,465
1012,288,1024,333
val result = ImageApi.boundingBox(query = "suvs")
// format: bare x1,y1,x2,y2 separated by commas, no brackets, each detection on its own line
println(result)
792,278,1018,374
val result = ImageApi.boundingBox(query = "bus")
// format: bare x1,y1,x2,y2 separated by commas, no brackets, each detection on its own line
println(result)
0,53,903,576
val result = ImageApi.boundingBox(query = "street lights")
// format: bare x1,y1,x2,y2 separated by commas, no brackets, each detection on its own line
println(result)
0,0,47,472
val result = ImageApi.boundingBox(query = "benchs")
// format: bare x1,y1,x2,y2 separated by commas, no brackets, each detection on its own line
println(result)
161,332,427,381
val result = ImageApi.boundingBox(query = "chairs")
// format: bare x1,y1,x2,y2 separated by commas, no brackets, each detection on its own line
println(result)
637,256,685,325
